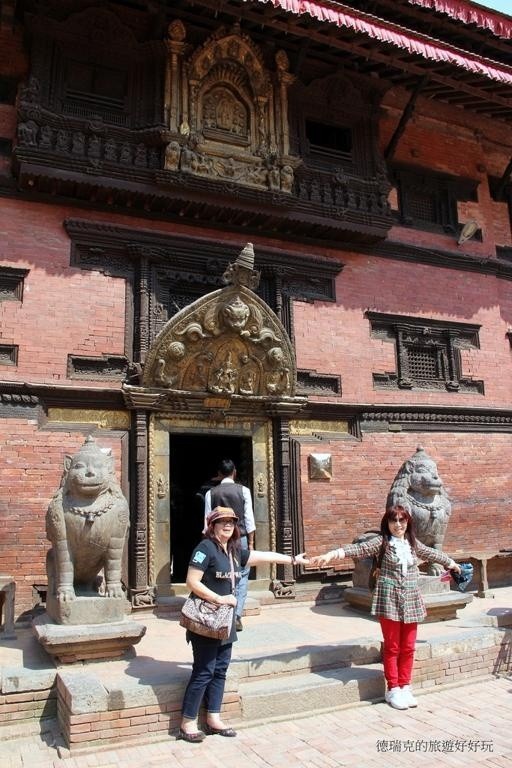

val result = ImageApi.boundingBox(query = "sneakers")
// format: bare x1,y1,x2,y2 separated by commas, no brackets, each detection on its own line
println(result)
235,614,243,632
384,685,409,710
401,684,419,709
178,726,207,743
205,722,238,738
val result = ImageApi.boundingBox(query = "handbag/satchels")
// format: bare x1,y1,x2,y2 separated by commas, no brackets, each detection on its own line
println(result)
178,589,235,641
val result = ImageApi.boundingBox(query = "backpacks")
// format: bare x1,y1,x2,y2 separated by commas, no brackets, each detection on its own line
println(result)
367,532,389,593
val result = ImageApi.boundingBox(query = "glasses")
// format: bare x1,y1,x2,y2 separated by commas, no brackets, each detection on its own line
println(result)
388,516,407,523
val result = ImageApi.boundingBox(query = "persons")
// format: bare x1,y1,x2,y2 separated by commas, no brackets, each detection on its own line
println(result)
178,504,312,743
201,459,258,632
307,505,463,711
194,464,223,530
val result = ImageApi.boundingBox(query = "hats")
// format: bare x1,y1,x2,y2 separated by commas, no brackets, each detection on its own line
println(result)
206,505,240,526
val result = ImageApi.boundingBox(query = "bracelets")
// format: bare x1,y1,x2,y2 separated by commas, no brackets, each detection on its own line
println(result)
291,554,297,565
248,540,255,544
